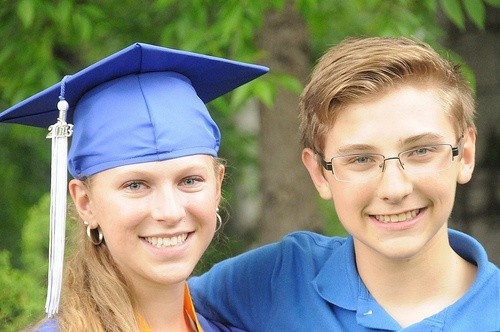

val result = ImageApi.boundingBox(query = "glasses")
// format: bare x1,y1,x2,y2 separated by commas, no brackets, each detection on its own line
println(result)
314,135,464,183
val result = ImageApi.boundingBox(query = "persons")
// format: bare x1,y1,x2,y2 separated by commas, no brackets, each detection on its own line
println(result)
12,88,246,332
183,35,500,332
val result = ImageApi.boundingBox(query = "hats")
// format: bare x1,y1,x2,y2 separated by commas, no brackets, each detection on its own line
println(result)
1,42,271,318
